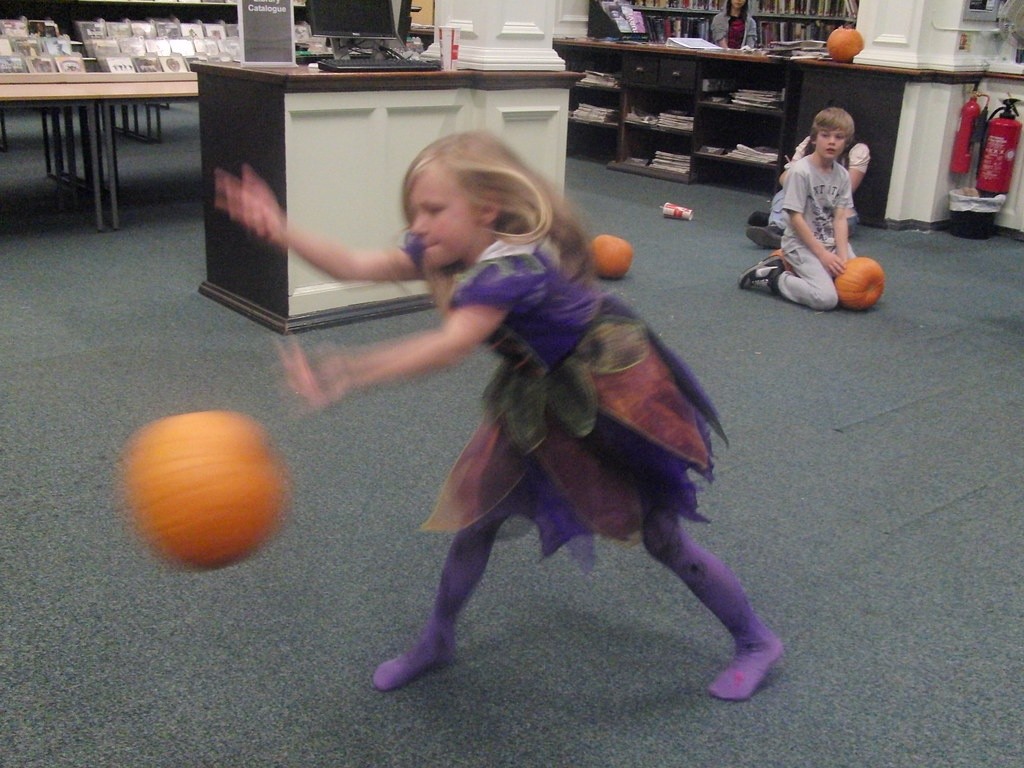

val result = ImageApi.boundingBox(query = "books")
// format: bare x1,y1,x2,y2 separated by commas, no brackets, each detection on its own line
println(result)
625,105,695,132
712,86,784,110
570,103,619,125
578,70,621,87
597,0,859,60
726,144,779,163
628,149,690,173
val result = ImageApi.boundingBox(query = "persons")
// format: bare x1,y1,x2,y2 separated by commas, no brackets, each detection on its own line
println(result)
737,106,856,311
746,99,872,248
213,129,783,704
710,0,757,49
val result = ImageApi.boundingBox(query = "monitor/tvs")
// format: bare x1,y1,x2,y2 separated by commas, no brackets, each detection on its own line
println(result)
306,0,397,60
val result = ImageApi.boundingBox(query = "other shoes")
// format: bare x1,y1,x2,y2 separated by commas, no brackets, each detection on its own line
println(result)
745,225,784,249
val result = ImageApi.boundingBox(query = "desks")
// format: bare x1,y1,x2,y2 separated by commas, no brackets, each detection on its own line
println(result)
190,59,586,337
0,82,203,233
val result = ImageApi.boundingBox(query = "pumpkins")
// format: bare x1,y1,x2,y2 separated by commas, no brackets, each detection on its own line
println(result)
123,409,289,569
834,256,885,311
591,234,632,278
828,21,865,63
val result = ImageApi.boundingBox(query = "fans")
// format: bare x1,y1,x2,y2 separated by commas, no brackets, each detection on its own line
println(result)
999,0,1024,51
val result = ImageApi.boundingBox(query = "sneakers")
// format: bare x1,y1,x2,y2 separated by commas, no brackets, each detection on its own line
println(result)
738,257,784,293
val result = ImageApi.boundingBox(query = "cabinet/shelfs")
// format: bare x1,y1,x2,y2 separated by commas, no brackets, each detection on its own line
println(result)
552,35,790,189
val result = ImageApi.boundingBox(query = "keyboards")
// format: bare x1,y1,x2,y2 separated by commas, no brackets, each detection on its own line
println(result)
318,59,440,73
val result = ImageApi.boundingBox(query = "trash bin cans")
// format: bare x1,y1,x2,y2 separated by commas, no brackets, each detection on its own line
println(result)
947,187,1009,240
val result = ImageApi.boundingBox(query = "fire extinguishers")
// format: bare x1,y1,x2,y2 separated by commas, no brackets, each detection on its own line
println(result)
976,97,1023,194
948,83,990,175
